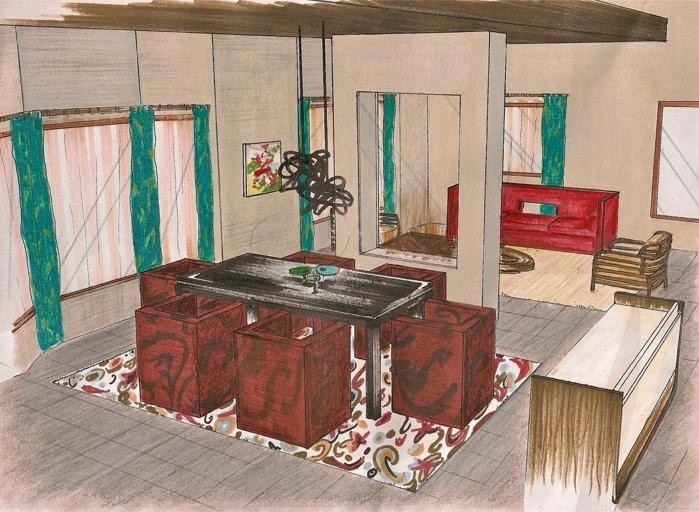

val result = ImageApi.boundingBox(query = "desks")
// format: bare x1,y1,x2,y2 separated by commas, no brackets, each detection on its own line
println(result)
174,252,432,421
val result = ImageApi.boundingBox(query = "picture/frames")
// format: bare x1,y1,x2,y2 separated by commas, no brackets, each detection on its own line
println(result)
649,99,699,223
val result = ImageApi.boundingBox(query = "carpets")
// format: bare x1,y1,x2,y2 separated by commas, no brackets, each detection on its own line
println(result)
52,336,542,496
492,244,643,313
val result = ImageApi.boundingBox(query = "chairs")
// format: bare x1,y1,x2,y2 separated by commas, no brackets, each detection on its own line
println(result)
587,228,672,296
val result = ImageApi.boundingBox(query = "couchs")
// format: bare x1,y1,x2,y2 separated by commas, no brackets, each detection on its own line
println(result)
440,179,620,258
